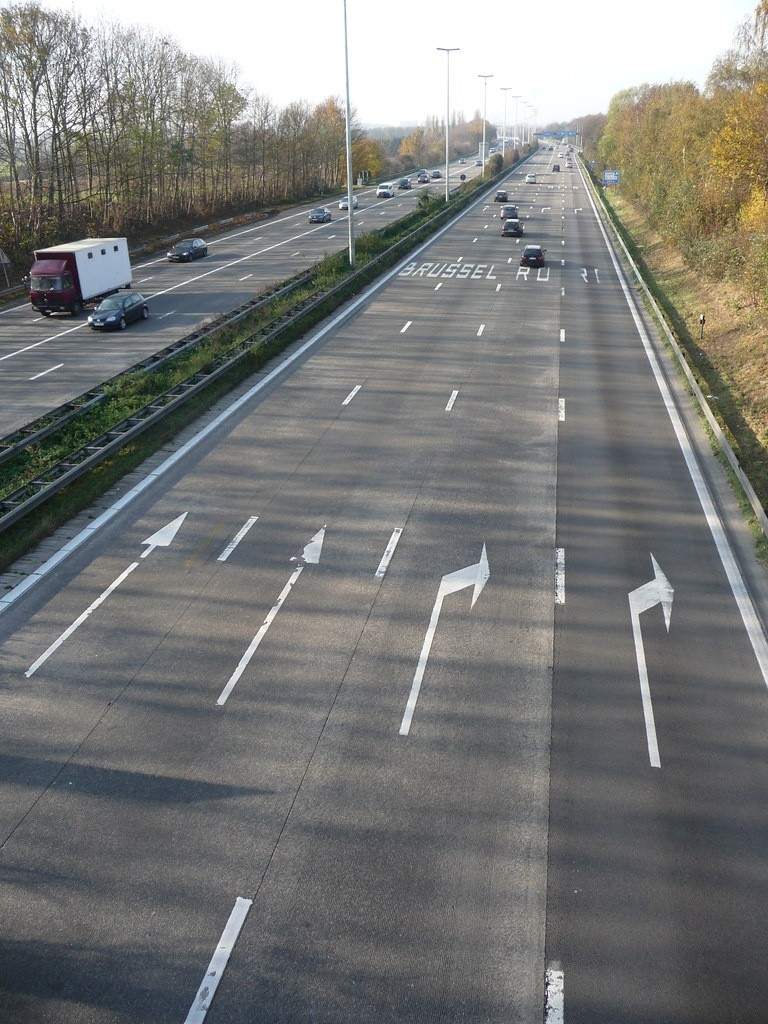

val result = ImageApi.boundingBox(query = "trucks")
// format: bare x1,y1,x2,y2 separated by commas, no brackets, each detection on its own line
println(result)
22,237,133,316
562,137,568,146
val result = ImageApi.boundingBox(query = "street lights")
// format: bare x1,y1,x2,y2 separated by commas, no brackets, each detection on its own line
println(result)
512,95,523,150
477,74,494,177
500,87,512,157
521,101,539,147
437,47,460,203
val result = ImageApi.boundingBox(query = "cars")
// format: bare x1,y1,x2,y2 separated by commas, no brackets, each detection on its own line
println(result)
308,207,331,224
87,292,150,331
417,169,429,177
542,141,573,158
167,238,208,263
501,219,524,237
494,190,508,202
398,178,411,190
475,161,482,166
417,174,430,183
525,173,536,184
566,157,572,162
458,158,466,164
431,170,440,178
552,164,560,172
565,162,572,168
490,148,495,153
338,196,358,210
500,205,519,220
520,245,547,267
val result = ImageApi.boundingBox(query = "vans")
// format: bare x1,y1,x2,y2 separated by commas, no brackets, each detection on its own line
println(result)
376,183,394,198
